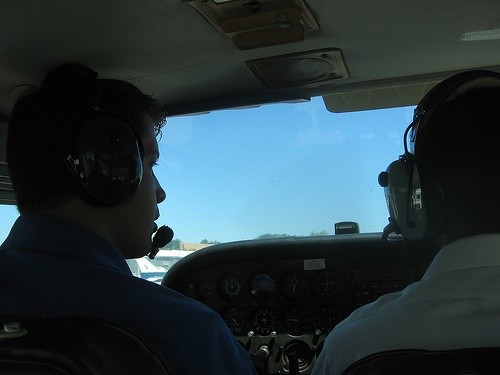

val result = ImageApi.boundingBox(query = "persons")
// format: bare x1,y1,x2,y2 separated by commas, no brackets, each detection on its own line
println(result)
0,78,256,375
311,84,500,375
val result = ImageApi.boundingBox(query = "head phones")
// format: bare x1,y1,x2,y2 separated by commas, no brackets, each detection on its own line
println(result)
378,69,500,242
56,66,144,207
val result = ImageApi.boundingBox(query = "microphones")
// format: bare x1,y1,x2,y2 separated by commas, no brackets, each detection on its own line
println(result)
147,225,174,259
